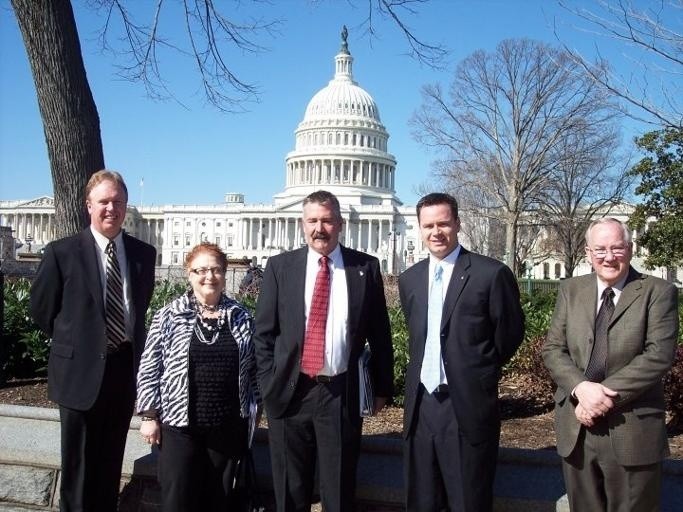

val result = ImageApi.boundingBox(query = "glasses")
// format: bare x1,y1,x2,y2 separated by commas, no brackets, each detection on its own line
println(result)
590,246,627,259
189,267,222,276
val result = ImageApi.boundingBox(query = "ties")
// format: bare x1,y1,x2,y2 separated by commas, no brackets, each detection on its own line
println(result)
583,287,614,383
105,240,125,352
300,256,331,380
419,265,443,395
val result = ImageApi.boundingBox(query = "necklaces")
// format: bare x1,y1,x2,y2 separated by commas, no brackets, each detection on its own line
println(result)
187,292,228,348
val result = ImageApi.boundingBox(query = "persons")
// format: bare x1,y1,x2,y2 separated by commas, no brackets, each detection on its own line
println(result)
134,241,264,510
394,191,525,509
253,190,398,510
539,217,680,511
27,168,159,509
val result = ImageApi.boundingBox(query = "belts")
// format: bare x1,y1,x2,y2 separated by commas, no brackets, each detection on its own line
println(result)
299,372,345,384
433,384,448,393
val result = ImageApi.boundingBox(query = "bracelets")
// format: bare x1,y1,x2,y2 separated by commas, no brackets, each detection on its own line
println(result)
139,416,156,422
